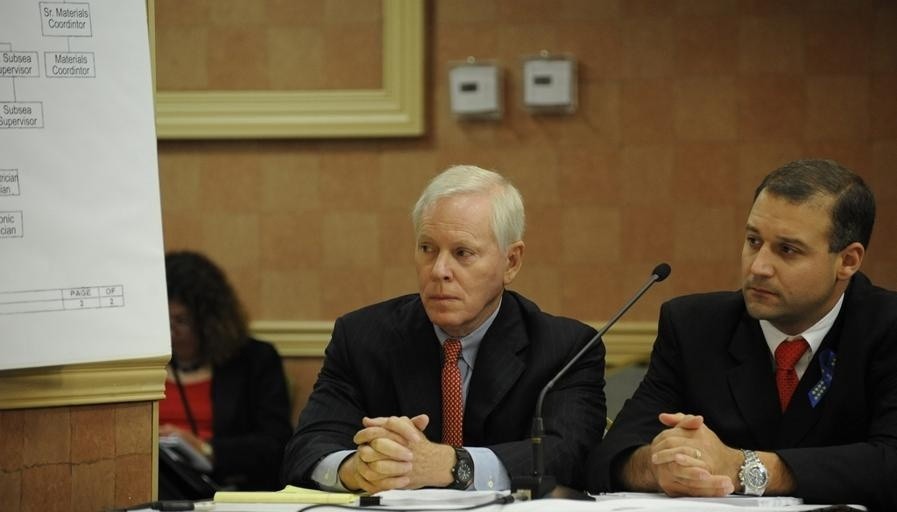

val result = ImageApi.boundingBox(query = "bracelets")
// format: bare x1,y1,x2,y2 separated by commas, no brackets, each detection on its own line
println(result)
200,440,213,462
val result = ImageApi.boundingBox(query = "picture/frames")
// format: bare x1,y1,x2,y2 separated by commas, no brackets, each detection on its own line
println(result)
144,0,429,144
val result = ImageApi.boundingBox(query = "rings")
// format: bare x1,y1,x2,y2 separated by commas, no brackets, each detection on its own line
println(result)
695,448,703,460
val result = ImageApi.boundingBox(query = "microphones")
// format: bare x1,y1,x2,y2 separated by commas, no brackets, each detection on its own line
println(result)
509,264,673,503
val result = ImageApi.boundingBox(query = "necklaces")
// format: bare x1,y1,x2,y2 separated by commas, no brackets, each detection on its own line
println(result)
169,355,209,375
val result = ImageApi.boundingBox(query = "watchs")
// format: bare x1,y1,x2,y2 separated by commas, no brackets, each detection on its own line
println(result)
445,444,474,490
738,447,768,496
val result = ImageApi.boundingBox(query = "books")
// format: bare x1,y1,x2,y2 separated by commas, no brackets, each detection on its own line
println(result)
159,437,213,475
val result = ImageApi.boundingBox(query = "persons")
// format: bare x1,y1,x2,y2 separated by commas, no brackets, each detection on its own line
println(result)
158,247,295,495
279,164,607,499
581,157,897,502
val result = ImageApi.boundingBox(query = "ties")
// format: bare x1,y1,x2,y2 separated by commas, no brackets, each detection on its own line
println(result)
437,337,465,448
772,336,810,415
